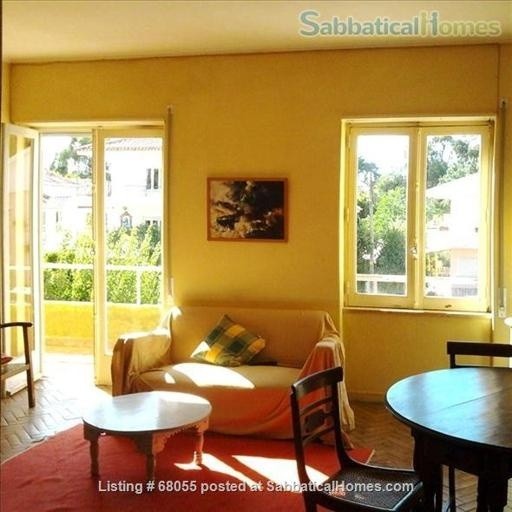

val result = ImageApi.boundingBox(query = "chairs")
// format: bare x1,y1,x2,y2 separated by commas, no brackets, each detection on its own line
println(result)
443,338,511,511
288,365,424,511
1,319,36,414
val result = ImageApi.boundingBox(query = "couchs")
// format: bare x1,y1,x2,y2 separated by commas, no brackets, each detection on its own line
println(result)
110,304,355,448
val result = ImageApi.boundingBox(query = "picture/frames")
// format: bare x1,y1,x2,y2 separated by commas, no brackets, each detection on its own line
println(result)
205,175,290,245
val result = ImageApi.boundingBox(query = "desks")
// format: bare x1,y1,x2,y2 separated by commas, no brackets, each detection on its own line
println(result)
382,368,511,511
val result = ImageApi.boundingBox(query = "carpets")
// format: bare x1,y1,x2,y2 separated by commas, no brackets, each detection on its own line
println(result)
2,423,373,511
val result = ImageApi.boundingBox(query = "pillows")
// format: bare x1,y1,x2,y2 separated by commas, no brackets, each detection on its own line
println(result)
189,312,268,367
